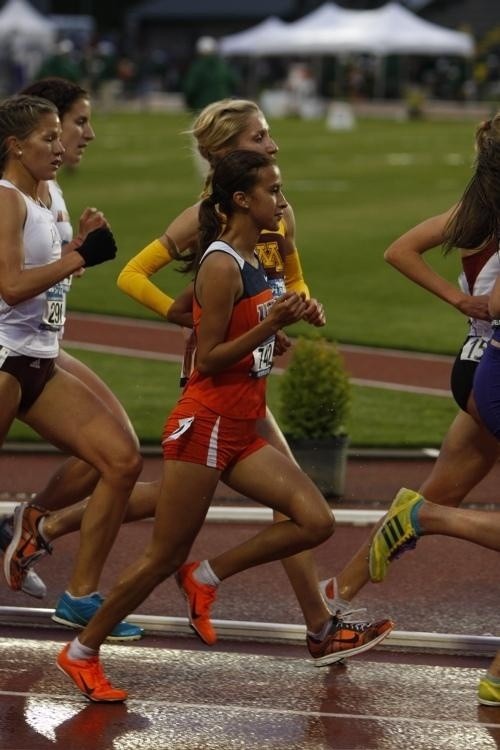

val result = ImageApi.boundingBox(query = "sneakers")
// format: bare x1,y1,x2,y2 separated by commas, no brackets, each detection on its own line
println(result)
173,560,217,646
3,501,53,592
305,607,395,667
477,676,500,706
0,515,47,600
50,590,145,641
366,486,425,583
55,642,127,702
317,577,353,623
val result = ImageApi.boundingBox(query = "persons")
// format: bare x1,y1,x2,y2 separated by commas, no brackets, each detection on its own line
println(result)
55,147,337,707
2,95,144,644
4,99,396,670
315,111,500,706
0,79,138,599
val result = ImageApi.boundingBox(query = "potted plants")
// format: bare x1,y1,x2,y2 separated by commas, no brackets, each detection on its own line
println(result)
277,329,351,496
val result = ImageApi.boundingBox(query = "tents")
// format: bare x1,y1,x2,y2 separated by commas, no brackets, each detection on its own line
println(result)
218,1,469,105
